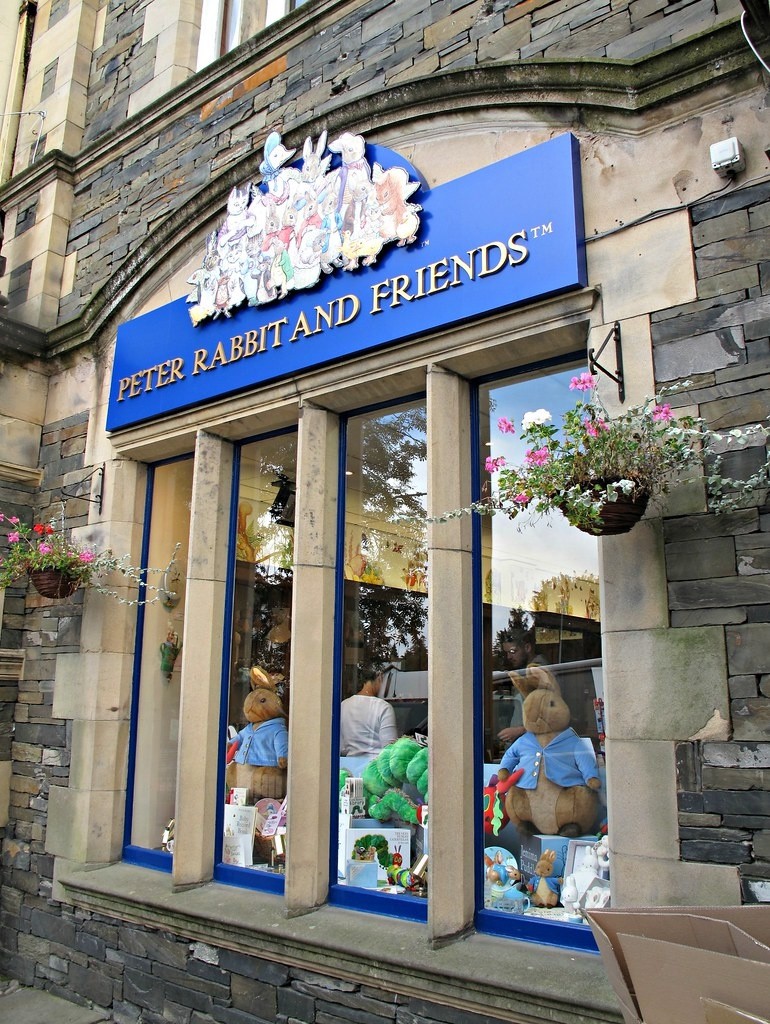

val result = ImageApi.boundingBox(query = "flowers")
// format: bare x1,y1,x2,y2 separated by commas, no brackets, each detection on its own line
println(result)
413,373,770,533
0,501,182,607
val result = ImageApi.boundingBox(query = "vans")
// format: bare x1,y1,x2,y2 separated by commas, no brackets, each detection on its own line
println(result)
404,658,606,765
377,662,428,741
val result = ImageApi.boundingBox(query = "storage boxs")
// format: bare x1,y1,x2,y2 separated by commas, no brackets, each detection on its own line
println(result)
346,826,411,887
519,832,599,904
346,860,378,888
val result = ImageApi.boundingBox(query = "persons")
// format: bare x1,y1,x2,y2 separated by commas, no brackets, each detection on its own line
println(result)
497,627,562,748
340,660,400,757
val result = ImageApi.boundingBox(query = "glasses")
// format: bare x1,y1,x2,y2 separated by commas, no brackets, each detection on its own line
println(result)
503,646,524,655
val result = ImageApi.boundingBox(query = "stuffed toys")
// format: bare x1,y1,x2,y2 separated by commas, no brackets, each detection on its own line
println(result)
527,846,598,915
497,664,603,838
225,665,289,806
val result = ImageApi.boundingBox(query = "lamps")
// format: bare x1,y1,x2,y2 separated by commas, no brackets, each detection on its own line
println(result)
268,471,295,528
